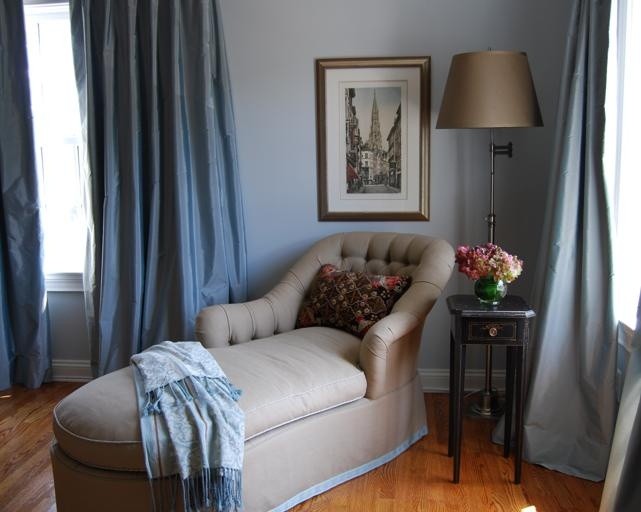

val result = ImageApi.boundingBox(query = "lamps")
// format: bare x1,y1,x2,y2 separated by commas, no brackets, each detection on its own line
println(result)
435,50,544,416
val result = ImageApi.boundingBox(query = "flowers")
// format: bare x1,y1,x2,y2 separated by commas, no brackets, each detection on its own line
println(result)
458,241,523,282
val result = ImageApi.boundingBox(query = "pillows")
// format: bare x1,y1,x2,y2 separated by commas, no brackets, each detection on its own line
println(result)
294,261,411,340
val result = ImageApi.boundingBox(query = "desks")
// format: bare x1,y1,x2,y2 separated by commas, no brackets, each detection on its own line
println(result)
448,296,536,486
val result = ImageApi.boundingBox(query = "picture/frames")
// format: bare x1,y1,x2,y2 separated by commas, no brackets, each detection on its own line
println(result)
312,57,431,223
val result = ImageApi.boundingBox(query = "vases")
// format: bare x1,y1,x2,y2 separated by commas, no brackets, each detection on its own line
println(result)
471,273,510,306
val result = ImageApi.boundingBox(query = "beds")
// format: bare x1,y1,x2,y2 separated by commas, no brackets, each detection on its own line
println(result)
50,229,457,512
